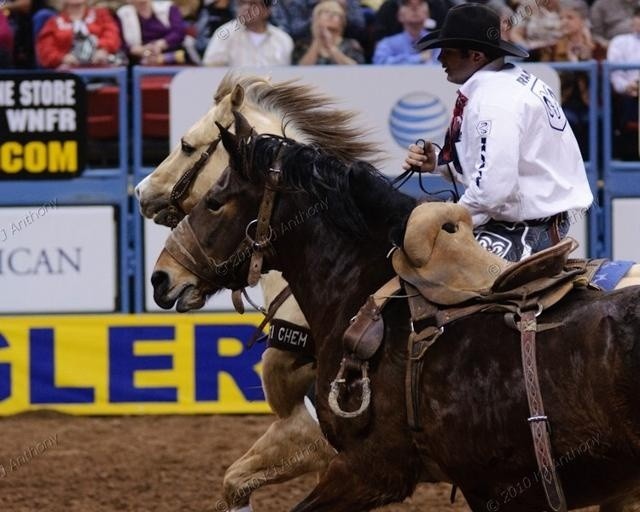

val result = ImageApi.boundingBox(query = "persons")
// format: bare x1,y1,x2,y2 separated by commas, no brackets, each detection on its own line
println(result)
399,3,595,266
1,1,639,165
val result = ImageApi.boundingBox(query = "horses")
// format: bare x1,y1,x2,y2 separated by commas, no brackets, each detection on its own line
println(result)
151,106,640,512
131,64,390,512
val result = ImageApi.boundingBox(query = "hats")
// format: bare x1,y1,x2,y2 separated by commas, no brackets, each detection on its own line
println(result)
411,3,530,57
